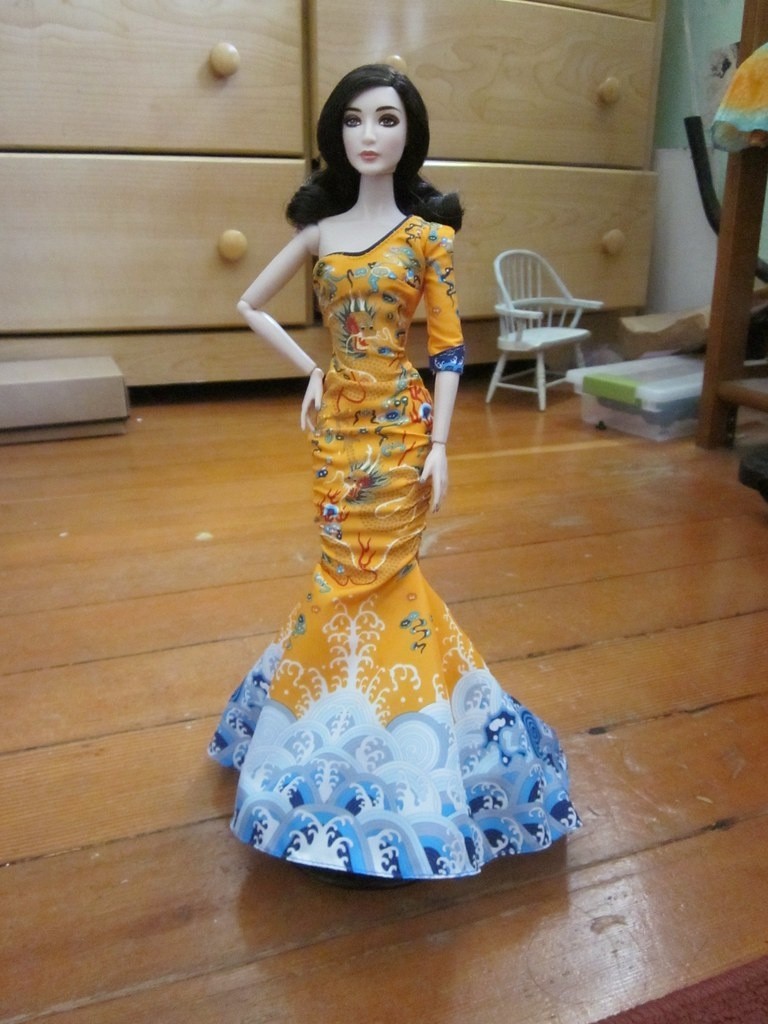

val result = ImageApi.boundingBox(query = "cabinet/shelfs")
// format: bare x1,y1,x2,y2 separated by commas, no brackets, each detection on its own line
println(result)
0,0,666,386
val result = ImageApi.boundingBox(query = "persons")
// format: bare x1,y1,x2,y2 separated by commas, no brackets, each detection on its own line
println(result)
202,64,578,880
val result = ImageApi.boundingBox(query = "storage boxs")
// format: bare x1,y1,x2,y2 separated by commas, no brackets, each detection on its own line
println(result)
565,355,705,441
0,357,131,445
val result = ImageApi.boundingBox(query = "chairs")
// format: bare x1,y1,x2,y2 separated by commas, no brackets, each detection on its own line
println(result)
486,248,603,413
